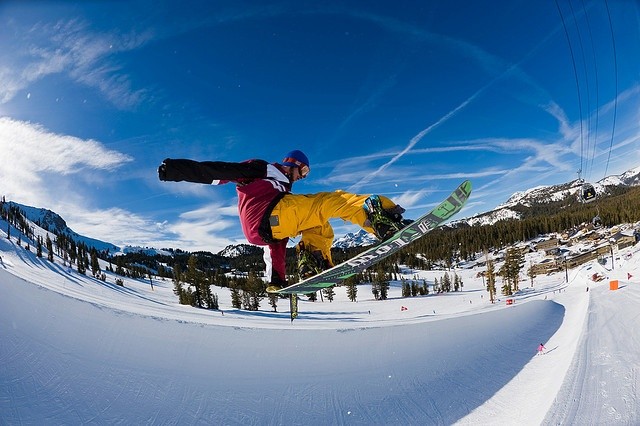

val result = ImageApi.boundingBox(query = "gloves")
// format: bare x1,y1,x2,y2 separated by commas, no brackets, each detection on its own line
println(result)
268,278,287,288
158,158,167,181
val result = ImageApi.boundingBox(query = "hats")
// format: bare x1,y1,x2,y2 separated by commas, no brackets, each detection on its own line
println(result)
282,150,309,169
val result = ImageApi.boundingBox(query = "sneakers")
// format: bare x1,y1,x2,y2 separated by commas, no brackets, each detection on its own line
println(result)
298,241,323,280
362,195,415,242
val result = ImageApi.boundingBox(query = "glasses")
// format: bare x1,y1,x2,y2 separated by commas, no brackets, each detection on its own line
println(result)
283,158,309,179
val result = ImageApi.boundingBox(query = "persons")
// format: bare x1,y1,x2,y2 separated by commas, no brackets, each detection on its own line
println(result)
158,149,406,287
536,343,544,354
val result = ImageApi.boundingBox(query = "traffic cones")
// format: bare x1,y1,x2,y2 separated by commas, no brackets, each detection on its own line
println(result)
610,280,619,290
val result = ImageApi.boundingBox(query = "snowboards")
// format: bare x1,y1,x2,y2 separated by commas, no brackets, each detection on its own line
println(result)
265,180,472,294
